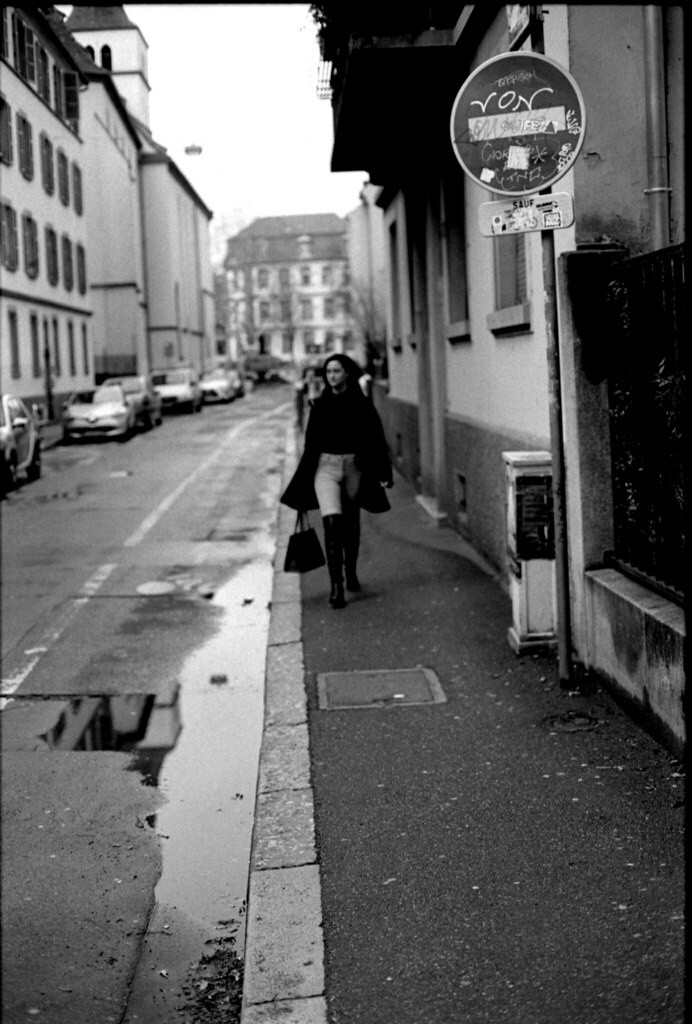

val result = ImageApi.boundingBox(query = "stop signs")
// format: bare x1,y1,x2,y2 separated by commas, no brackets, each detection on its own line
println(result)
450,50,587,195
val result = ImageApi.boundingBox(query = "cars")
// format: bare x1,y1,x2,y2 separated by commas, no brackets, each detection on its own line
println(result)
0,395,44,490
60,385,137,441
151,366,204,414
197,354,262,402
101,375,164,429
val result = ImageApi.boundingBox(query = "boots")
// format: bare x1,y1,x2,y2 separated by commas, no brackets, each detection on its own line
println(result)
322,514,345,607
344,511,362,593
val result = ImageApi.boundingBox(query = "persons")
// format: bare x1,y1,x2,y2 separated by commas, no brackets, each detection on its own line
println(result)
280,354,393,607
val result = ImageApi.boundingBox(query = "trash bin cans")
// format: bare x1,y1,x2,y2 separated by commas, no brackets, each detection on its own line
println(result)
502,450,558,656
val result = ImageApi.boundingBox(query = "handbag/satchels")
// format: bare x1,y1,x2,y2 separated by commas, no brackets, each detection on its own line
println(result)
283,511,325,574
